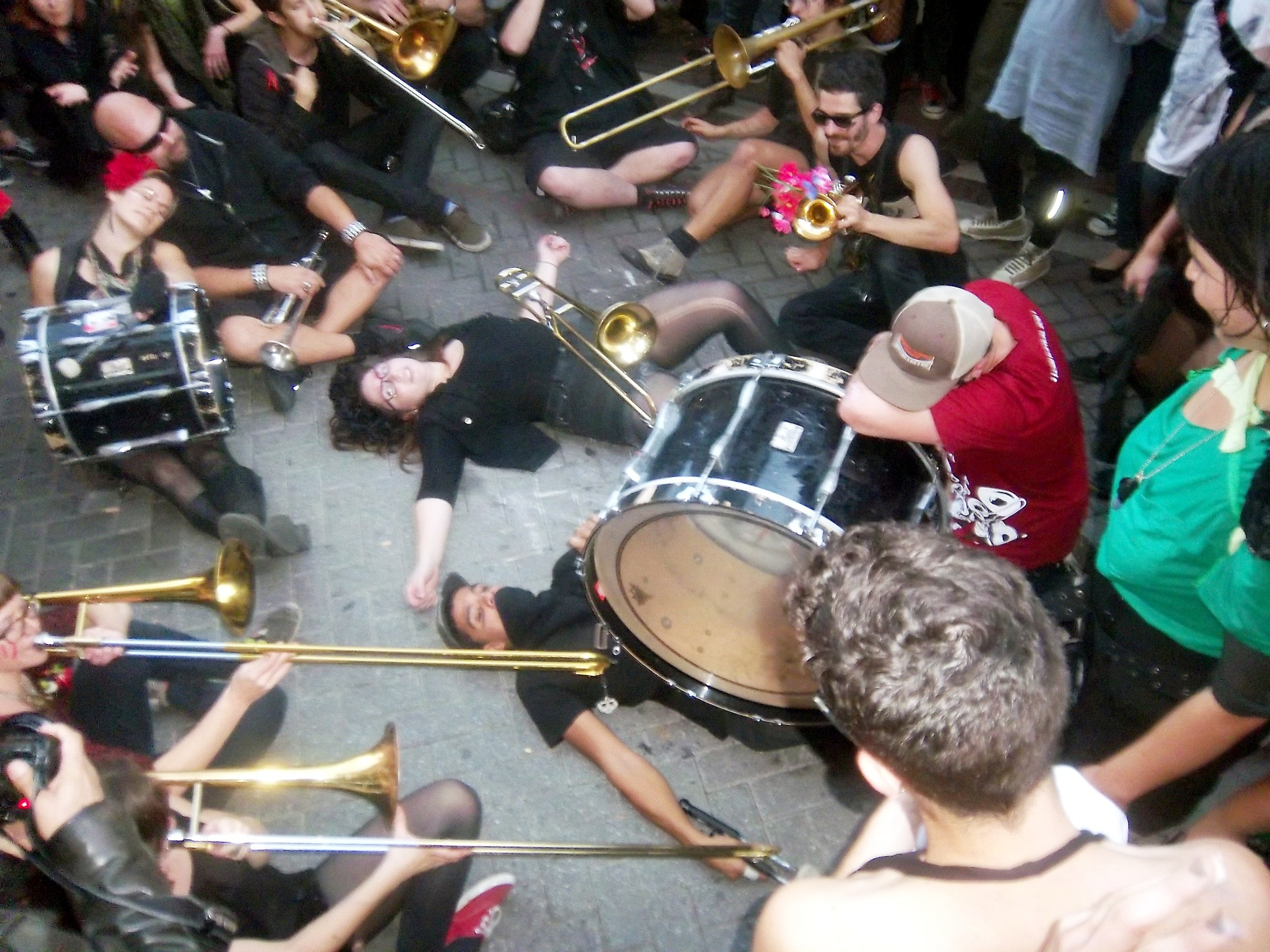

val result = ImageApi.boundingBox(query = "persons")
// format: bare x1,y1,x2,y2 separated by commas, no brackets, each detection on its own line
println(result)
755,518,1269,952
331,234,797,611
435,512,808,882
1185,774,1269,843
840,278,1085,599
0,0,508,559
0,573,516,952
1060,119,1270,839
490,0,993,363
955,0,1270,494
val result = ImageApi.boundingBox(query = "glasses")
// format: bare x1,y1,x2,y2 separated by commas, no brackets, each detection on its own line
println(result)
374,361,397,410
119,103,168,155
1,599,39,645
812,106,869,129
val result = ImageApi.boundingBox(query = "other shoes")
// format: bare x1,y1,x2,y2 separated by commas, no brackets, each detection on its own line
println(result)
1086,266,1124,282
621,234,685,286
1070,351,1109,382
255,603,301,644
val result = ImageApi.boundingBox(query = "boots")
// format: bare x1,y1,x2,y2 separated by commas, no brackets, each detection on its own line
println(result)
639,180,693,211
187,462,311,558
550,198,576,223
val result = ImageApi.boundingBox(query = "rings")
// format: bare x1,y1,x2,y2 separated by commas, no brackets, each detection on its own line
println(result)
395,256,399,261
304,282,311,292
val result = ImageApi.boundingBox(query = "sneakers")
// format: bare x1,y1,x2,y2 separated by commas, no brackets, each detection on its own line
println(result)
363,310,438,351
991,238,1054,290
441,206,492,251
922,82,946,120
377,216,444,252
959,205,1029,242
266,364,307,412
0,137,52,186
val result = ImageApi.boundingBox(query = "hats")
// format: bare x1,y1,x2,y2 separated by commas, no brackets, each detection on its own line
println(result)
856,285,994,413
436,572,471,648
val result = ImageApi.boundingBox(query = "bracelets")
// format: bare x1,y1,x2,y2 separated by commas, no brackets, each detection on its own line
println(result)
340,222,367,245
537,261,556,268
251,263,272,292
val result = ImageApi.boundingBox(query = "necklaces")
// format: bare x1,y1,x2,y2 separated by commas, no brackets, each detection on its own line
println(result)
179,158,214,201
1112,352,1269,509
86,246,142,294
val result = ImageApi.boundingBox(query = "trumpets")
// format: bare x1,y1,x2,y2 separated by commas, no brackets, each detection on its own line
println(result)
257,229,331,372
792,174,863,240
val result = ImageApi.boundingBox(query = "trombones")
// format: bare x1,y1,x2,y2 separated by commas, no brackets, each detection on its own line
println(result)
557,0,891,152
311,0,486,150
138,722,782,857
494,265,659,428
21,538,611,670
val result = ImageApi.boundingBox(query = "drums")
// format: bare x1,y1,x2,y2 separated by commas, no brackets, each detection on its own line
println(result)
582,354,946,726
16,282,236,469
